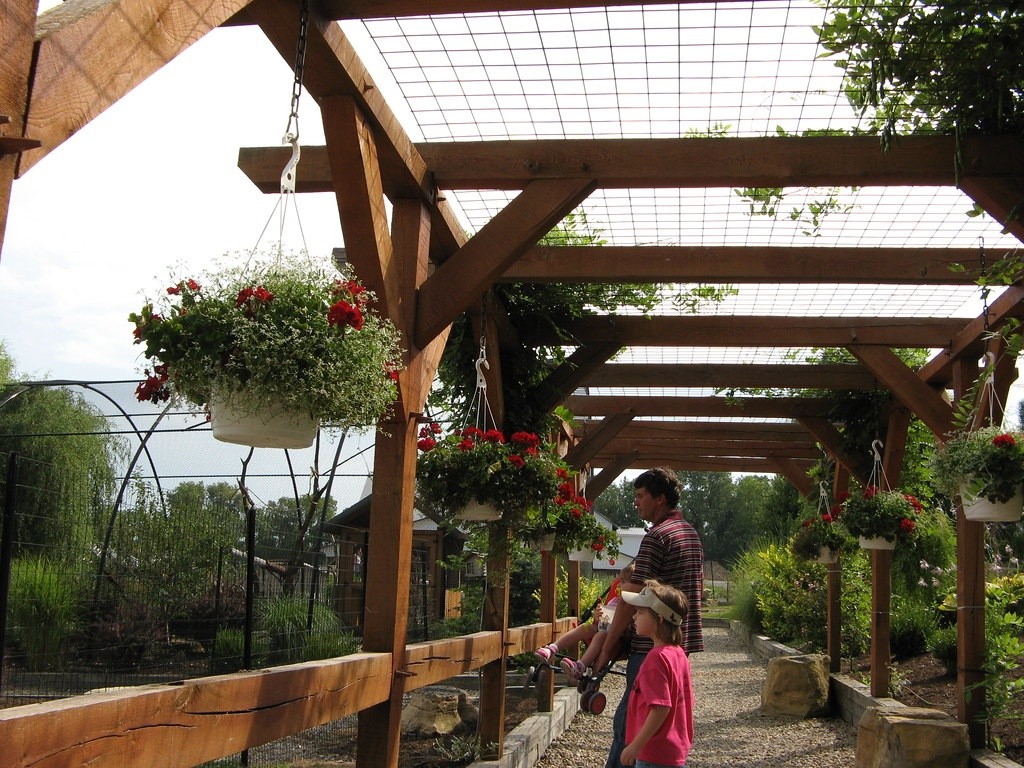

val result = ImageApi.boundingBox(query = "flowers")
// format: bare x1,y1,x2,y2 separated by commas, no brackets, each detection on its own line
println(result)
841,485,923,544
793,514,845,561
928,426,1024,504
128,242,624,565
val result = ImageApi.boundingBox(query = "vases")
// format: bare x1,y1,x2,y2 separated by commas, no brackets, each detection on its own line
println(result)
530,532,556,551
211,387,316,449
454,498,503,521
959,483,1023,521
568,540,595,561
858,535,897,550
808,545,839,563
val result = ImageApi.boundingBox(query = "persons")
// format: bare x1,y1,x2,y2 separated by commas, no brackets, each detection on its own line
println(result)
591,467,705,768
621,580,695,768
533,563,635,680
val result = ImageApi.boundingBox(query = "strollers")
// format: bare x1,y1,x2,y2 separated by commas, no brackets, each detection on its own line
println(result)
531,556,637,715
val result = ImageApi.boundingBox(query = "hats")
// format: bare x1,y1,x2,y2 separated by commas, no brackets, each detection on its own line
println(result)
621,586,683,626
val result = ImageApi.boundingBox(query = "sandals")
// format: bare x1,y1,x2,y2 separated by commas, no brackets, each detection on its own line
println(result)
560,659,582,684
534,645,555,665
